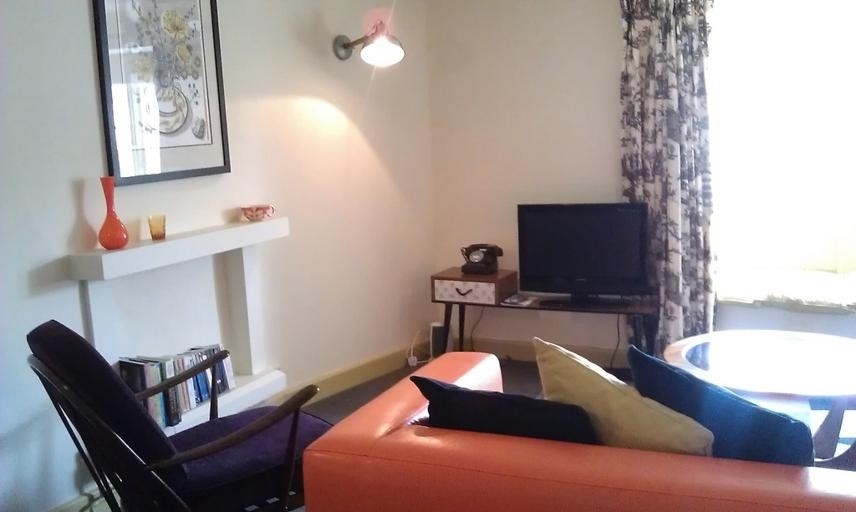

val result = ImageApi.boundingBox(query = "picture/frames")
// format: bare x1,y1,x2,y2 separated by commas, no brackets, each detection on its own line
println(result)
92,0,231,188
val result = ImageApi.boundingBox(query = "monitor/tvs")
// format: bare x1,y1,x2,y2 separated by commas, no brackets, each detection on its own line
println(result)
517,203,650,311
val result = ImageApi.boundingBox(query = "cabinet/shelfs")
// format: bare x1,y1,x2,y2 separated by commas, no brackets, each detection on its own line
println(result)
431,267,517,304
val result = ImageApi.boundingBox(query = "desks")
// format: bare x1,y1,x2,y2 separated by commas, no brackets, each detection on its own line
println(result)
432,292,658,356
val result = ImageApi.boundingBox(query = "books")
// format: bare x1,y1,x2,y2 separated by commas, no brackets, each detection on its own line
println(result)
116,342,237,432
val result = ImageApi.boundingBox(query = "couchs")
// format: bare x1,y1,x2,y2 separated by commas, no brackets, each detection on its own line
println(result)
303,350,856,512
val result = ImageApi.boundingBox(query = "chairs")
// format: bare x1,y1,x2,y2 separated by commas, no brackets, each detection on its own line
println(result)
26,319,334,512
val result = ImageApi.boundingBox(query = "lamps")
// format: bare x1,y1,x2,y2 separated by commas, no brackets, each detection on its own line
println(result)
333,19,406,68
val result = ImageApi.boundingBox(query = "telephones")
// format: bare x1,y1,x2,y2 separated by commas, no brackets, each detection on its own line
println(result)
461,243,504,274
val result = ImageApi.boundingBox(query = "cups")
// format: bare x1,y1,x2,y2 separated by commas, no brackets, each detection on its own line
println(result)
148,215,167,241
241,203,276,222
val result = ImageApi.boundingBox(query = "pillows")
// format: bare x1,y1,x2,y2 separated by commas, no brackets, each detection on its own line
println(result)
531,336,715,458
409,374,596,445
626,345,815,468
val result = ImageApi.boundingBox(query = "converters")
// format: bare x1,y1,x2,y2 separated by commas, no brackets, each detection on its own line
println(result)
408,355,418,367
